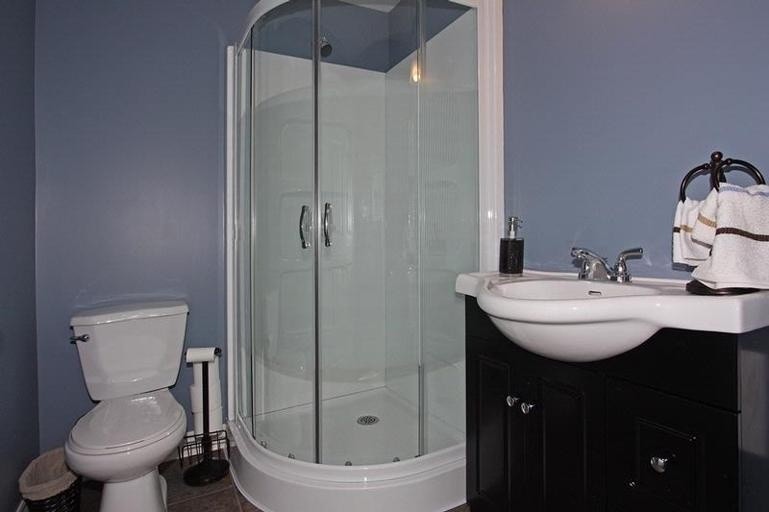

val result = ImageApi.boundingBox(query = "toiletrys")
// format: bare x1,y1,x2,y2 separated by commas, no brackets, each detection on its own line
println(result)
498,214,525,273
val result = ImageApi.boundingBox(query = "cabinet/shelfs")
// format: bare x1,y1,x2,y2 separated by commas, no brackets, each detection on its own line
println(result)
607,376,740,512
466,334,606,511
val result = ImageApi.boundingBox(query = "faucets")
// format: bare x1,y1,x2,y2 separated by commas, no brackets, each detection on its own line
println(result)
570,247,614,276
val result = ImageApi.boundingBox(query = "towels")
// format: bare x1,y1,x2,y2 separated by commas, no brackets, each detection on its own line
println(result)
670,182,769,291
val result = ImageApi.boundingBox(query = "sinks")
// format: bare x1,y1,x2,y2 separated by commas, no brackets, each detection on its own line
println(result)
456,267,769,363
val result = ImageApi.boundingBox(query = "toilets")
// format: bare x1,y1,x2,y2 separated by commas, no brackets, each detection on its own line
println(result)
65,299,189,511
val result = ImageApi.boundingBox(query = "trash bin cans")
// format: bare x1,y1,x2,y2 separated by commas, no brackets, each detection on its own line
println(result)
18,446,83,512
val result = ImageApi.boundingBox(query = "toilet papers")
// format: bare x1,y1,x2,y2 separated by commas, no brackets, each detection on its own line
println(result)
187,345,223,436
